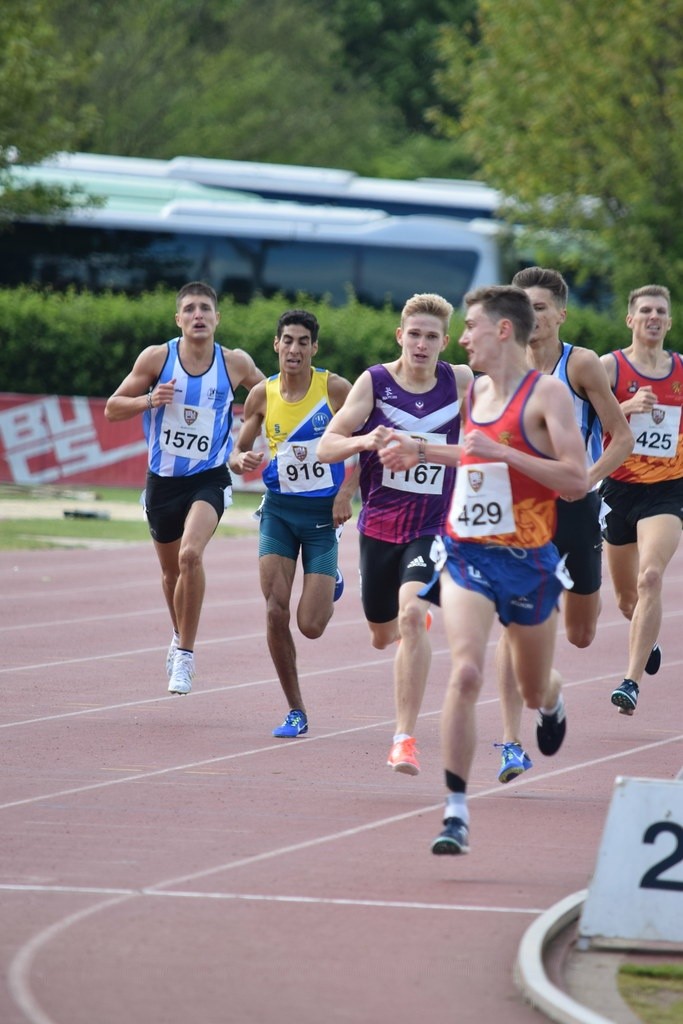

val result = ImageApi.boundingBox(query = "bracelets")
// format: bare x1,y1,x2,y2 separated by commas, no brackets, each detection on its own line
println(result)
419,443,427,463
147,393,154,408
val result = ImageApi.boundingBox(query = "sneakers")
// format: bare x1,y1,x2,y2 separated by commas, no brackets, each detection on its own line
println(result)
494,741,532,784
386,736,422,776
645,643,661,675
165,635,181,678
397,609,433,648
333,566,344,602
610,677,639,716
272,709,308,738
168,653,196,695
536,698,567,757
430,817,470,854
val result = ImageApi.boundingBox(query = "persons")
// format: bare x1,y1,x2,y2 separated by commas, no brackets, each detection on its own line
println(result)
600,285,683,716
378,286,588,855
228,311,361,740
499,267,634,783
104,283,266,695
318,293,473,775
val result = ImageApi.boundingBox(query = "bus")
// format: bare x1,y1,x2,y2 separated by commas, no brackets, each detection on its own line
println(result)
0,162,618,317
41,149,618,228
